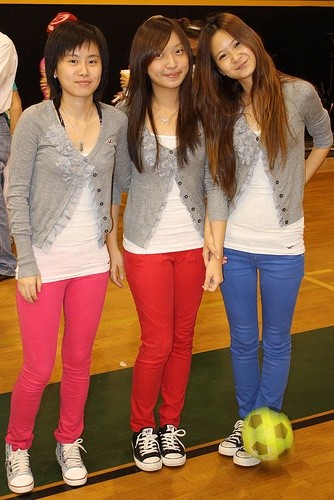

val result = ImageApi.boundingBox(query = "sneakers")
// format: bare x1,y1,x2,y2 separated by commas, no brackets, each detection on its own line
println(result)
131,426,163,472
4,442,35,494
156,423,187,467
55,438,89,487
233,445,263,467
219,420,245,456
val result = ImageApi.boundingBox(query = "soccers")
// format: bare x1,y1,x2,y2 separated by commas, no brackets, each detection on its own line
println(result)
240,406,294,463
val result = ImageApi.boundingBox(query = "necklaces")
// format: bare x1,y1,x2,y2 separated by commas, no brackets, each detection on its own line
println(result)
249,112,259,129
152,107,179,125
60,108,94,151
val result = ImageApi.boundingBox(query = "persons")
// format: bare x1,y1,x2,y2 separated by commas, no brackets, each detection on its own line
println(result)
0,11,213,282
191,13,334,467
3,19,131,493
107,15,228,471
